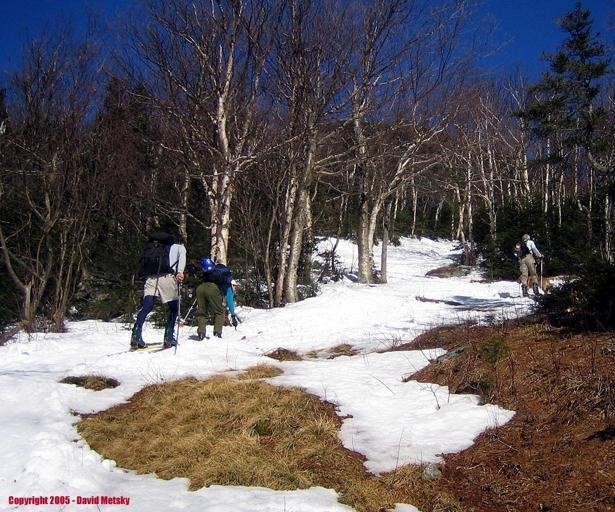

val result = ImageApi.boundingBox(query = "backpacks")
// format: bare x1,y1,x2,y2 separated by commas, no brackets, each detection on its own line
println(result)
203,264,231,285
513,241,528,258
142,231,174,275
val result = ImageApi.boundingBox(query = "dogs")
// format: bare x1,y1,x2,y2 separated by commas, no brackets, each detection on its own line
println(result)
517,274,553,296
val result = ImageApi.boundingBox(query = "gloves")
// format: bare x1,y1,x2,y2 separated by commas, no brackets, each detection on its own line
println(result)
232,319,237,326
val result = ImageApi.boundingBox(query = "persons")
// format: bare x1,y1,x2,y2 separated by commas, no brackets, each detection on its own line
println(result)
195,264,236,340
513,234,544,297
131,235,187,349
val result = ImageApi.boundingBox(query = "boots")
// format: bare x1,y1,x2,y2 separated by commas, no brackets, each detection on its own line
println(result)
164,327,176,348
130,323,145,349
522,285,529,297
214,332,222,338
198,332,210,340
533,283,539,295
